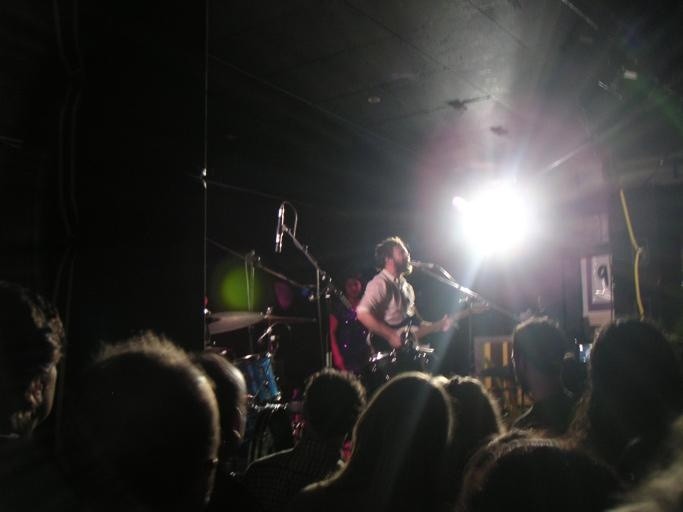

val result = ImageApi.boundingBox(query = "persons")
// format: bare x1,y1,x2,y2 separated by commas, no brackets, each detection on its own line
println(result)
326,270,363,369
0,281,683,512
354,235,459,356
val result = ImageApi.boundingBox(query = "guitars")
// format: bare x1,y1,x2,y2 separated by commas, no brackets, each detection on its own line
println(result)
371,302,490,360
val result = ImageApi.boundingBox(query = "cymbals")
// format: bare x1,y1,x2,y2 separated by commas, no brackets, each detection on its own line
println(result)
206,310,264,338
261,315,318,326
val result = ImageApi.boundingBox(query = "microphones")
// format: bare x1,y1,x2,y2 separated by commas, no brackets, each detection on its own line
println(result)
274,205,286,254
410,261,433,270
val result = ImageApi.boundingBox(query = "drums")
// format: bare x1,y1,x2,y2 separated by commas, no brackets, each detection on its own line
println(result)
232,347,284,407
369,344,433,386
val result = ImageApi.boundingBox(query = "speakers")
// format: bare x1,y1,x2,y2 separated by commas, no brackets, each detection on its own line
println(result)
608,182,683,338
